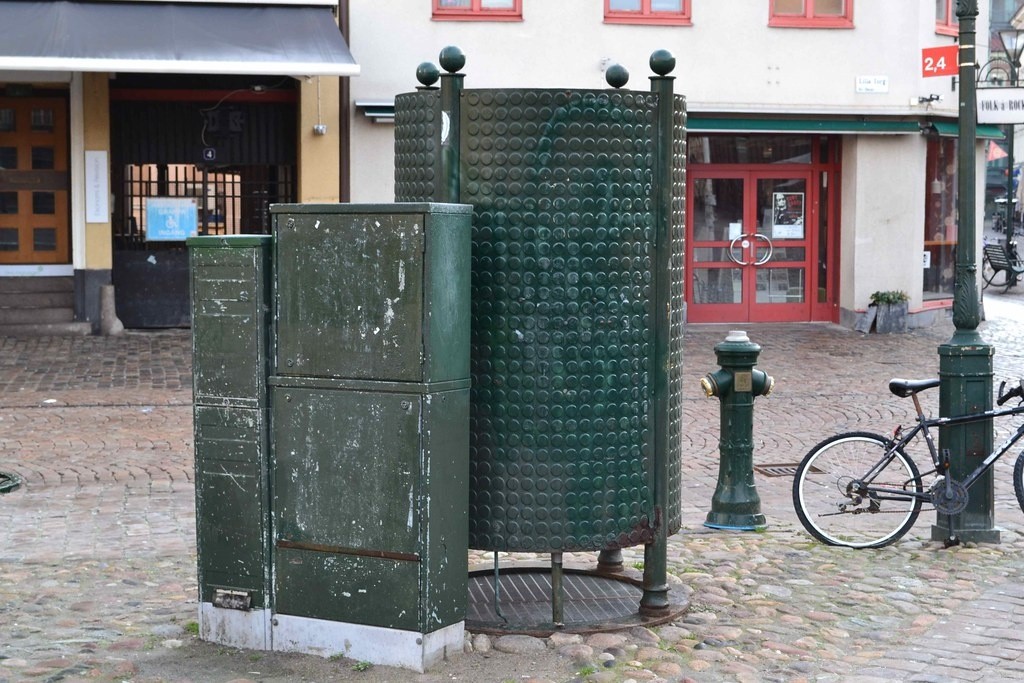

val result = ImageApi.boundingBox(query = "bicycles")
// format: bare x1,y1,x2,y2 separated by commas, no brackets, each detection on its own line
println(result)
983,233,1024,287
792,376,1024,550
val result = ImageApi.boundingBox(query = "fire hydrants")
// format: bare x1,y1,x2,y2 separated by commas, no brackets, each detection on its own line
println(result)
699,330,775,532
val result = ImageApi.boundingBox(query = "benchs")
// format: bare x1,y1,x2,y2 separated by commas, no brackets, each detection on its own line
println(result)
983,243,1024,293
